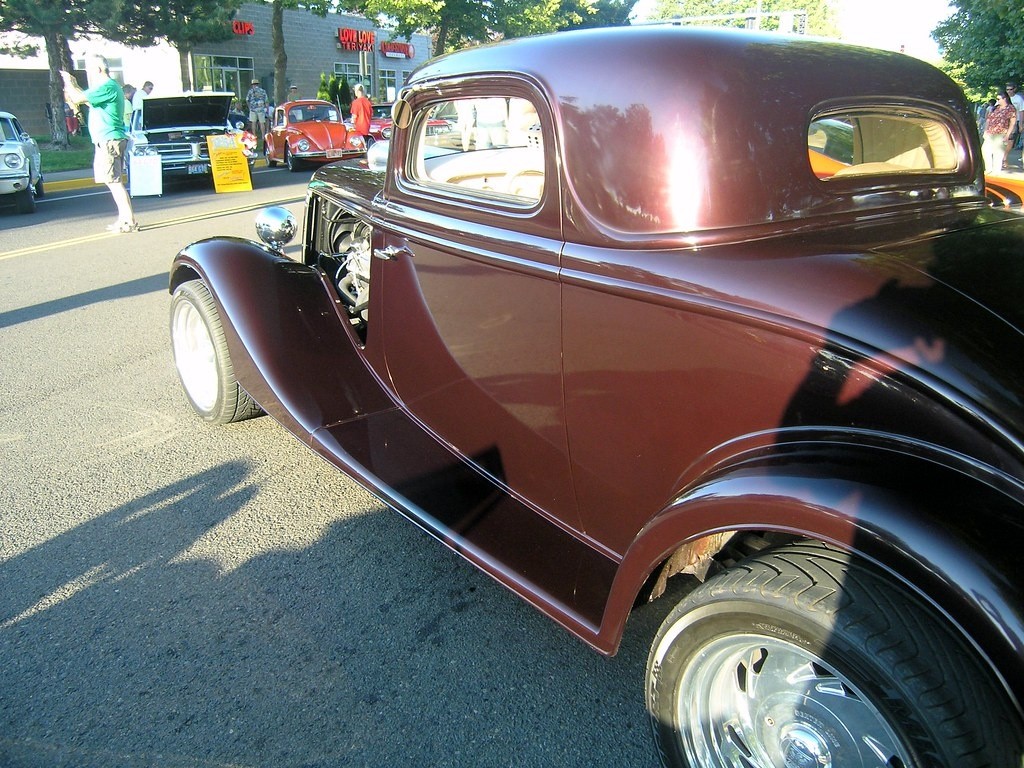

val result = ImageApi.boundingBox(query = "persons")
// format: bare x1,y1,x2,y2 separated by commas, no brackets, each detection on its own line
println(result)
454,98,475,152
287,86,303,101
981,90,1017,177
508,97,541,147
976,99,999,136
121,84,134,174
245,79,269,139
132,81,153,130
232,102,245,116
1002,82,1024,172
350,84,373,158
59,55,139,233
474,98,510,150
48,90,85,141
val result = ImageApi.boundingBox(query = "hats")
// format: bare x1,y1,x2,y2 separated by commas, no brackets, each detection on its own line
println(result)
250,80,260,85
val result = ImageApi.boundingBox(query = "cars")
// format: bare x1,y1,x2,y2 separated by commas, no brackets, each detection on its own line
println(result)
163,23,1024,768
119,91,256,179
341,102,455,140
808,116,1023,205
263,98,366,172
0,111,43,210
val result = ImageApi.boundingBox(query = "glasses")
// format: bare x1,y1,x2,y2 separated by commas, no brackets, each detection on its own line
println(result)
147,86,152,90
291,87,297,89
1006,88,1013,91
995,98,1000,101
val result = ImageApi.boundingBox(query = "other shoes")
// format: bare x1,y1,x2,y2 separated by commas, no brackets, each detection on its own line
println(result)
107,220,140,233
1002,159,1008,170
